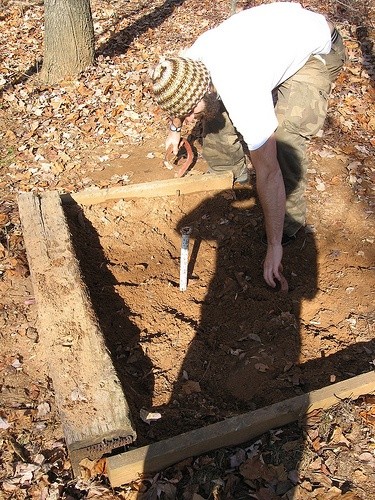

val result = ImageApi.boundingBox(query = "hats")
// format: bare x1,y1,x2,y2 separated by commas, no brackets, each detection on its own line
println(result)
152,56,211,122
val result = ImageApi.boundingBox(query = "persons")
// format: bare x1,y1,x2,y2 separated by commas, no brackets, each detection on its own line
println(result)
148,0,346,287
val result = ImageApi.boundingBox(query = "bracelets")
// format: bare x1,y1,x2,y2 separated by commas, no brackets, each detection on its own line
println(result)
170,124,181,132
